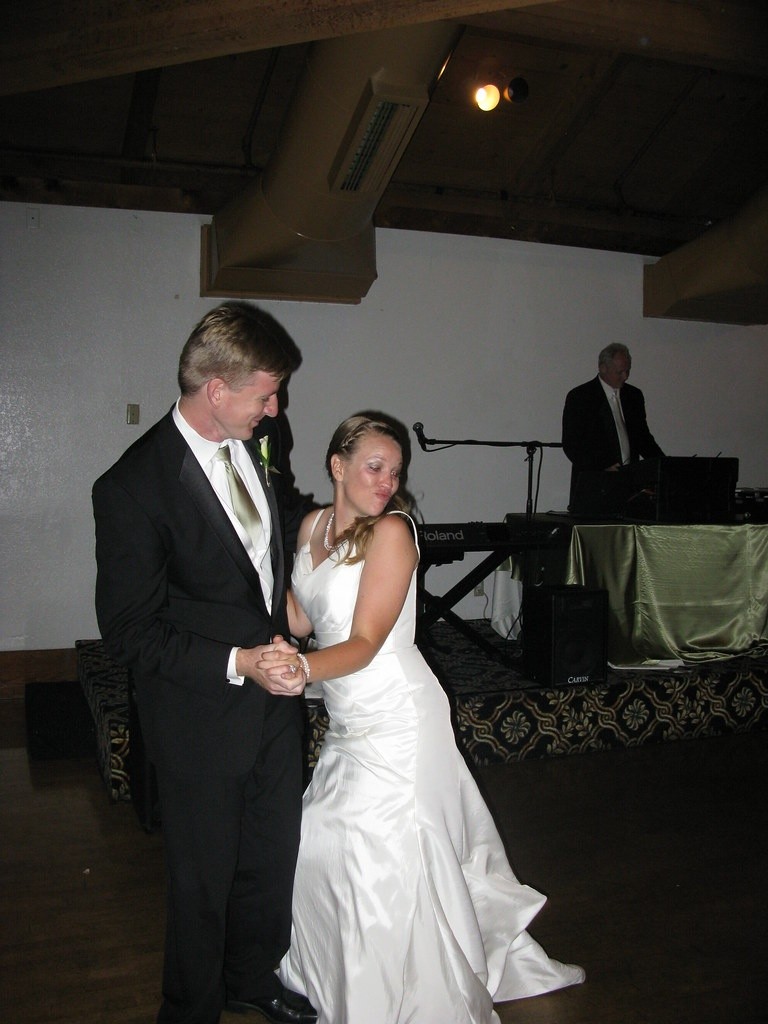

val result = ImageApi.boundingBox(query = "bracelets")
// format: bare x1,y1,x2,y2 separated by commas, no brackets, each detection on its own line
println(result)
296,653,310,681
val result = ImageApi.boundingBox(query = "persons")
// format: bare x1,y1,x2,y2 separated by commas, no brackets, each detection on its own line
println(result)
256,414,583,1022
562,343,664,514
90,301,315,1023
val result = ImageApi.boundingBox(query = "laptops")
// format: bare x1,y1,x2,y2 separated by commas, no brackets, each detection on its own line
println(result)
545,471,632,518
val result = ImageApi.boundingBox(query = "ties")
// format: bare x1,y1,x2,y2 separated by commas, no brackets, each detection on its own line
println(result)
215,446,263,547
612,396,630,464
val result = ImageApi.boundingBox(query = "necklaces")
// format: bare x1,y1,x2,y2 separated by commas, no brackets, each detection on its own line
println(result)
324,512,349,552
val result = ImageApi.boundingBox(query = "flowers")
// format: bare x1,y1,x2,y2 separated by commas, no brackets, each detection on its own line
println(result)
252,436,280,487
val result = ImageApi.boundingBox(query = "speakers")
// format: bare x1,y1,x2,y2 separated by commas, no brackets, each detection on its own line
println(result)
126,666,162,835
520,585,610,687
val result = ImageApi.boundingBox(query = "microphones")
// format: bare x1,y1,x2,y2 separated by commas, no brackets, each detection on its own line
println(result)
413,423,426,452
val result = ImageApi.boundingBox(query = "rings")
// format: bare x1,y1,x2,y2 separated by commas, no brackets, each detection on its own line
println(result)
288,664,296,674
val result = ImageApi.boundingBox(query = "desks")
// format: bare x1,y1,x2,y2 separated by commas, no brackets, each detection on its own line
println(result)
506,512,768,669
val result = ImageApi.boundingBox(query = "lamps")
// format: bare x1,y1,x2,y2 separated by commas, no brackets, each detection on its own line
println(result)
504,77,529,103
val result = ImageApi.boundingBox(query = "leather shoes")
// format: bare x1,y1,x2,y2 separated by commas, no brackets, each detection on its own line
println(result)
227,986,316,1024
